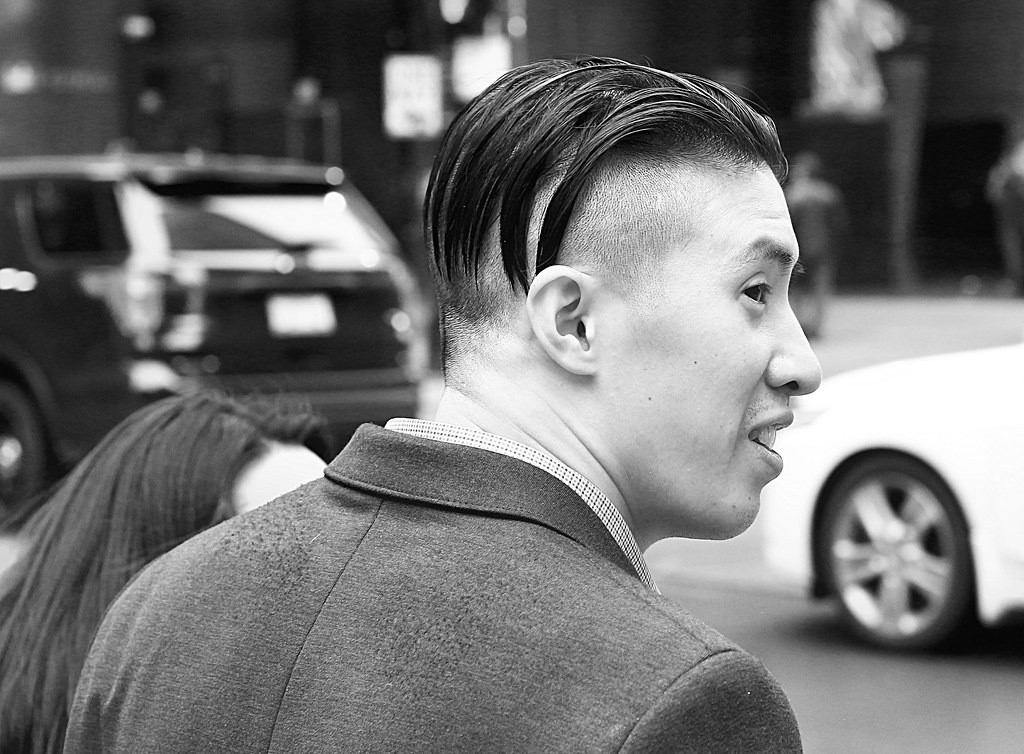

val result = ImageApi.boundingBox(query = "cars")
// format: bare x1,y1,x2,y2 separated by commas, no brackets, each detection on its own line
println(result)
760,342,1024,655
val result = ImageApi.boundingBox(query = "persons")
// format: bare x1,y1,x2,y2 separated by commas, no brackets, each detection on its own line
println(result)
0,385,329,753
57,53,823,754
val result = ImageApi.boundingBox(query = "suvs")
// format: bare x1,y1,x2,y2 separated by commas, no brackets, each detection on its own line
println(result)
1,149,422,536
